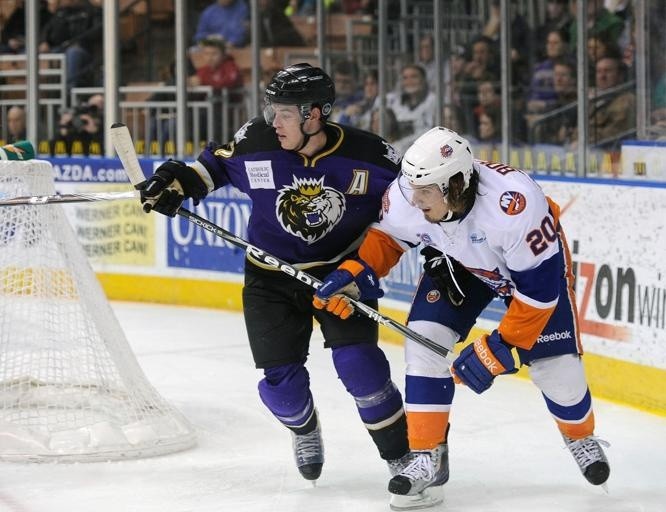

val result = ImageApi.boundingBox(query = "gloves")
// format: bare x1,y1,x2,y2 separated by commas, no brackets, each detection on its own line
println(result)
311,257,386,323
140,159,204,217
449,329,522,394
424,258,472,309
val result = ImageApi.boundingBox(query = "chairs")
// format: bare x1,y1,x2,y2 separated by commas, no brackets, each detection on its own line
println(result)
0,0,665,167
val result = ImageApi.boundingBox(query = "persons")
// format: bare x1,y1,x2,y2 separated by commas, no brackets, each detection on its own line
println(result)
0,1,666,153
312,126,612,493
135,61,410,480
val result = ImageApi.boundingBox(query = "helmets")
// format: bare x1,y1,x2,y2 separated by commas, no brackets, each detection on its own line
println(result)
394,126,478,209
262,63,337,128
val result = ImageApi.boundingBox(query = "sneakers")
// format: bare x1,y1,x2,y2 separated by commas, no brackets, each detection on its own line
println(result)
561,434,610,486
387,442,449,502
292,427,324,480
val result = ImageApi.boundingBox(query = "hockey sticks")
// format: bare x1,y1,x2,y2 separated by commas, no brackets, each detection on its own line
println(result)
110,121,453,361
